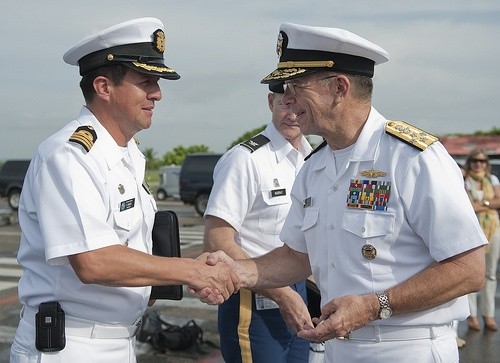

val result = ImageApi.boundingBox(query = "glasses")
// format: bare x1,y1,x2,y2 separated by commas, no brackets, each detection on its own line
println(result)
270,94,290,109
471,159,487,164
283,76,338,99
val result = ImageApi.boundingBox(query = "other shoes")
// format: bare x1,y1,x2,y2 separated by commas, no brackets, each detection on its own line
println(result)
483,316,498,330
456,338,465,348
468,316,480,331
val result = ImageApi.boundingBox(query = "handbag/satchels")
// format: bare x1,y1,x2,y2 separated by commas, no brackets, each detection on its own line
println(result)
136,308,202,354
150,211,183,300
304,278,321,326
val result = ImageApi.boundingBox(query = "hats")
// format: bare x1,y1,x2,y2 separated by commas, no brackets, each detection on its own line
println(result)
260,23,389,83
269,82,284,93
63,18,180,79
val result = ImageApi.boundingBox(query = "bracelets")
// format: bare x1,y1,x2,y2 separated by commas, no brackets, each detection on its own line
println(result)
483,200,490,207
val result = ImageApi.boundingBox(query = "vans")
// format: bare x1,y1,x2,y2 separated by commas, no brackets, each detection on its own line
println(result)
157,164,183,200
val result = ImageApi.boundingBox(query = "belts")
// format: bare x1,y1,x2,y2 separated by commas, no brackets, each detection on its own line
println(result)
334,323,453,341
22,305,142,339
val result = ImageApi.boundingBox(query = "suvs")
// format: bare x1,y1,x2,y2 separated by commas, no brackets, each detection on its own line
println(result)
180,151,225,216
0,159,32,210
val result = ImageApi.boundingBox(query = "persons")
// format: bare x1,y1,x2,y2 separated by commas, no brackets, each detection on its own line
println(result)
189,15,500,363
10,16,242,363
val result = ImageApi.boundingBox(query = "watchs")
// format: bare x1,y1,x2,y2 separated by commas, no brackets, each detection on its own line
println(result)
374,291,392,320
